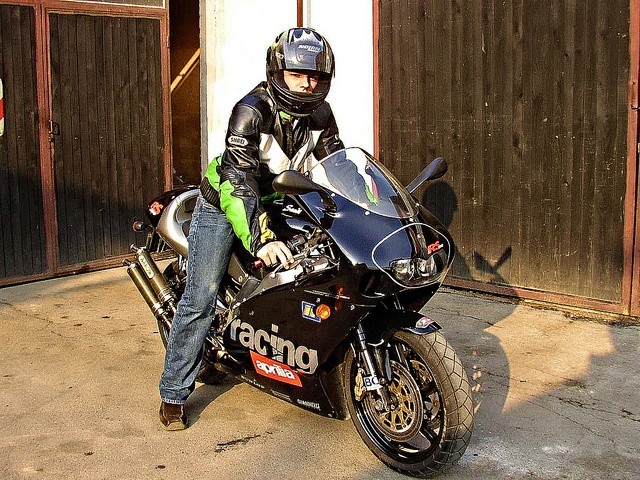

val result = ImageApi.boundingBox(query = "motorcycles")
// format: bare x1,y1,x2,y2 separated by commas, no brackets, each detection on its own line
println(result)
122,147,475,478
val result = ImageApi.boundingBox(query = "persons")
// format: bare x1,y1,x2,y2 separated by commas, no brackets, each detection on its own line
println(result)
157,26,379,431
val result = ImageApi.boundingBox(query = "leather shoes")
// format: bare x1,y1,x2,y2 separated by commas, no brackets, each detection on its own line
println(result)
157,401,188,432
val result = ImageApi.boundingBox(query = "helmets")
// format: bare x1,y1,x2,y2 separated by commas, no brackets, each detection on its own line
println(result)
265,24,336,120
